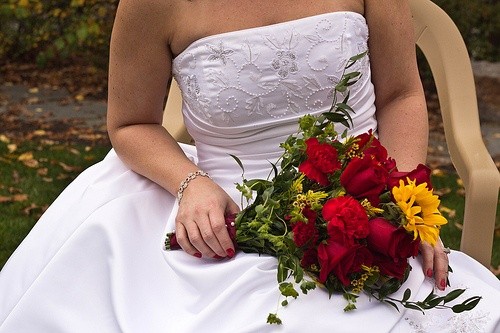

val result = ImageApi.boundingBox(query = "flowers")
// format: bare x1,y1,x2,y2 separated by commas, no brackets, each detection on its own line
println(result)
163,50,485,327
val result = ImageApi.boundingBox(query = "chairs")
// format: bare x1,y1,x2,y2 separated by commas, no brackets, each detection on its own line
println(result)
162,0,500,275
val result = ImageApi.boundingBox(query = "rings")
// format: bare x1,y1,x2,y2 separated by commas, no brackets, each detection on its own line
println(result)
443,247,451,255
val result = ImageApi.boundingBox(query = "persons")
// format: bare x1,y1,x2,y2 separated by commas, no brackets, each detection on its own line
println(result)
0,0,500,333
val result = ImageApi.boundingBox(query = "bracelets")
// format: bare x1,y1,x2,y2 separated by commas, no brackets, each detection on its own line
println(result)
175,170,213,206
